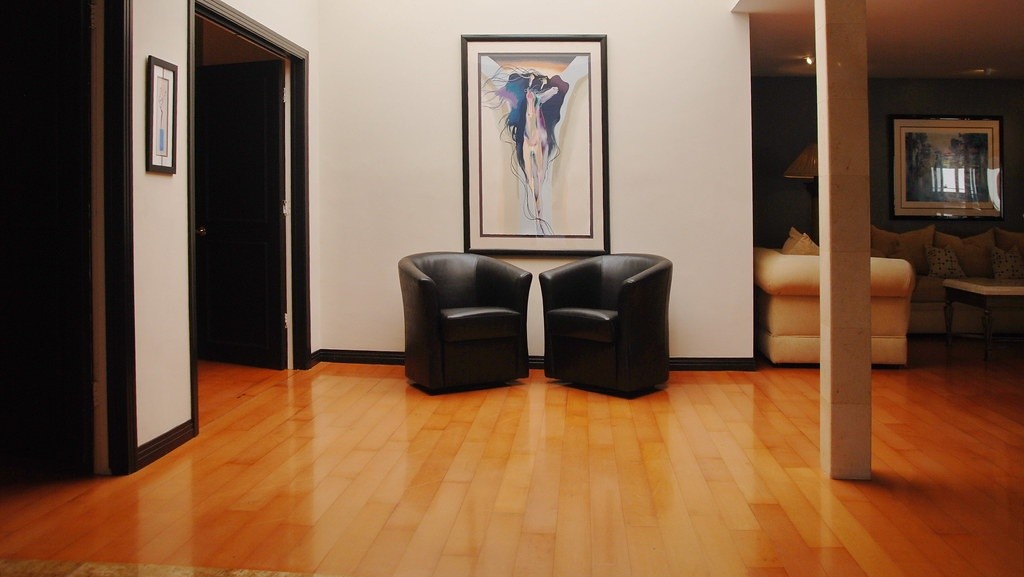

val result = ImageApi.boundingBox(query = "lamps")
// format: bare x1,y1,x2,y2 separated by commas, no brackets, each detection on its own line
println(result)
782,143,819,248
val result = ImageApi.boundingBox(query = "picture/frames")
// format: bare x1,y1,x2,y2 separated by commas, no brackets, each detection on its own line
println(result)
886,112,1006,223
460,34,613,259
146,54,179,174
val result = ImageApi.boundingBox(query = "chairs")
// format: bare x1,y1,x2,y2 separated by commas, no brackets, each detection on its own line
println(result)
397,251,534,396
539,253,675,396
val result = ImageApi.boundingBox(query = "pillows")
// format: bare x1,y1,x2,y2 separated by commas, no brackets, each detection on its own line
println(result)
776,227,819,255
870,225,1024,284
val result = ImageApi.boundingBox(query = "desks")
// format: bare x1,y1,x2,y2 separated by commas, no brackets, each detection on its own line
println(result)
942,278,1024,362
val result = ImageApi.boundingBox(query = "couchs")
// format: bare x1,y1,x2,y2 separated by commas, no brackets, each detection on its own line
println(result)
753,241,917,369
870,223,1024,344
